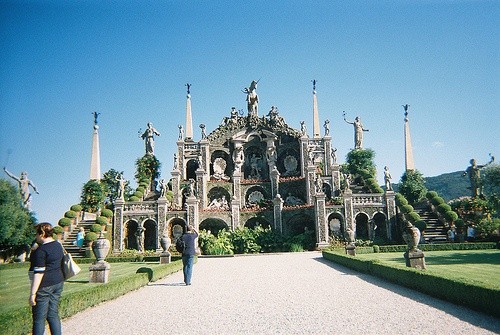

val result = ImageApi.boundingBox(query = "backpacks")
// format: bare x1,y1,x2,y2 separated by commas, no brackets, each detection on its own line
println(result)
175,233,186,254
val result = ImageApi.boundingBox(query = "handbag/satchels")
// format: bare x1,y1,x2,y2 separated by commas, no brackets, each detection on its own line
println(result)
56,240,81,281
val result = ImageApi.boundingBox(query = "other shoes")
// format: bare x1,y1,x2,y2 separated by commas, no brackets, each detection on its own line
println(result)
186,283,191,286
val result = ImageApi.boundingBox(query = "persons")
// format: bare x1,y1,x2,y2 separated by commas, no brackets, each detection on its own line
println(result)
315,173,323,193
114,173,128,199
231,107,237,124
250,154,263,178
331,148,338,164
383,166,393,191
178,225,198,285
178,125,183,141
344,175,352,192
207,195,228,209
446,221,477,243
300,121,307,134
4,167,39,209
174,153,178,170
344,116,370,149
187,178,197,199
461,156,495,198
324,120,330,135
266,106,287,128
135,226,146,252
198,124,206,139
159,179,169,198
18,223,87,335
140,122,160,153
286,193,303,204
